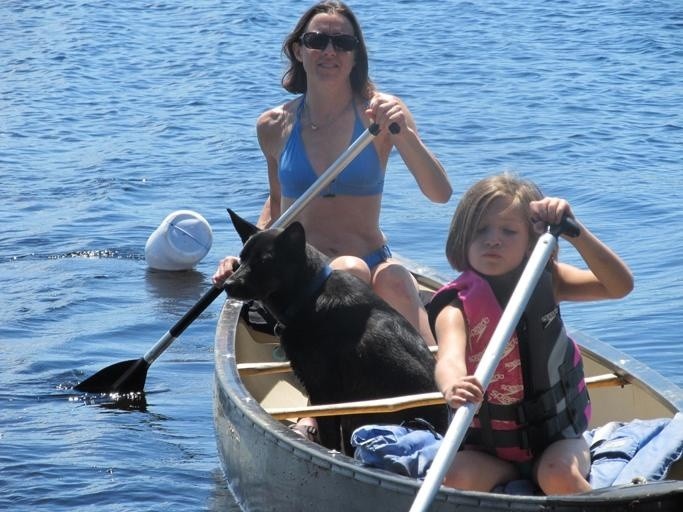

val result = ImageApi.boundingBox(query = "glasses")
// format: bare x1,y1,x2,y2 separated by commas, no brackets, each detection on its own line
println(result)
298,31,357,51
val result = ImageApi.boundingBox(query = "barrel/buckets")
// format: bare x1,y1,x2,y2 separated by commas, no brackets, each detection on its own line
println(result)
144,210,212,270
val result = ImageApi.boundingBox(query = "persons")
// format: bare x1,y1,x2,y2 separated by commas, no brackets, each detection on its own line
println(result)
211,2,437,441
427,175,633,498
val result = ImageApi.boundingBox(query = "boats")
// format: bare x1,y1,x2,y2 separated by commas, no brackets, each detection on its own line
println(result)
215,252,682,512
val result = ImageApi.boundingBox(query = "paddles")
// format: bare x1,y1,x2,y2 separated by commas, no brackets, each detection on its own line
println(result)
73,100,400,394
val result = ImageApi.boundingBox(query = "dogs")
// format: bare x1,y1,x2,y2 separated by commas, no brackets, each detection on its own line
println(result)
221,207,468,459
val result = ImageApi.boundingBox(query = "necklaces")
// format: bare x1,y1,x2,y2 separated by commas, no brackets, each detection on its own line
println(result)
305,101,352,131
